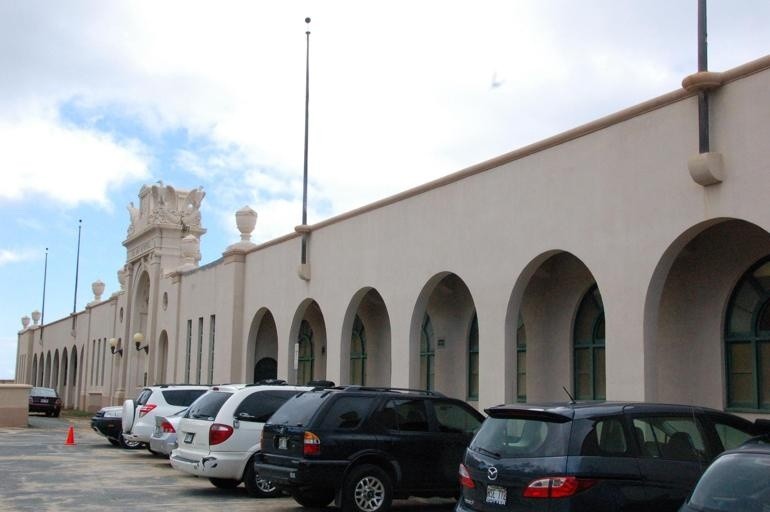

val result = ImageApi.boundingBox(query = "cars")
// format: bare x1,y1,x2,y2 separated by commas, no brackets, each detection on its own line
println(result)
89,406,144,449
149,408,188,454
28,384,61,418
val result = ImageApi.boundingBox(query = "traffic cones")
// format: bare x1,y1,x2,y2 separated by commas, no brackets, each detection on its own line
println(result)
65,423,76,445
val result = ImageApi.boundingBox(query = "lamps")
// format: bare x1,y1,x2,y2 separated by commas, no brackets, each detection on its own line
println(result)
109,338,123,358
134,333,148,355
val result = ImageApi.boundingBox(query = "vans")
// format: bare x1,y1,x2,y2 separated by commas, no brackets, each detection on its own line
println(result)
454,384,768,511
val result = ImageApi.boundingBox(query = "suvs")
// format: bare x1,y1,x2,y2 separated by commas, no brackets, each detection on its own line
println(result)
167,380,335,497
123,381,211,457
256,384,487,511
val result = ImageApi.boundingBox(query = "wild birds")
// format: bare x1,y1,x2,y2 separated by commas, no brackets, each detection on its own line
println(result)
151,179,178,210
126,201,139,222
182,184,207,210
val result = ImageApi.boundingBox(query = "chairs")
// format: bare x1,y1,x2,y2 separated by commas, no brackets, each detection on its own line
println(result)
518,422,698,456
